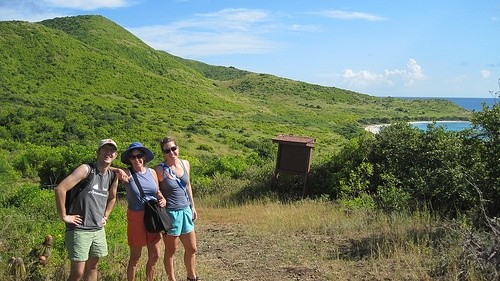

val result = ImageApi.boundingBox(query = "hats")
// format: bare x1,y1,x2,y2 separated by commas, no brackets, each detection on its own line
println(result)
121,143,154,165
98,139,117,152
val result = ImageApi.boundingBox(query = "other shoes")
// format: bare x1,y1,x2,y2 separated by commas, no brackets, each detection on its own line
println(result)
187,276,201,281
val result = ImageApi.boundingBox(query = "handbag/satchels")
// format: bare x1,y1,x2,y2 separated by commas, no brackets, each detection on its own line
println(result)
144,200,171,233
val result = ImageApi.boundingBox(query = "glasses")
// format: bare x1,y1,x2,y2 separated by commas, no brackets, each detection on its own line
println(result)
129,154,142,160
164,144,178,154
101,147,116,152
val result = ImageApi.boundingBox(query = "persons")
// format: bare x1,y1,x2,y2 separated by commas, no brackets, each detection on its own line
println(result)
55,139,118,281
108,142,166,281
154,137,203,281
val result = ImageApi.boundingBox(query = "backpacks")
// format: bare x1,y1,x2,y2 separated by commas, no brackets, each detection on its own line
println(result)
60,162,116,214
